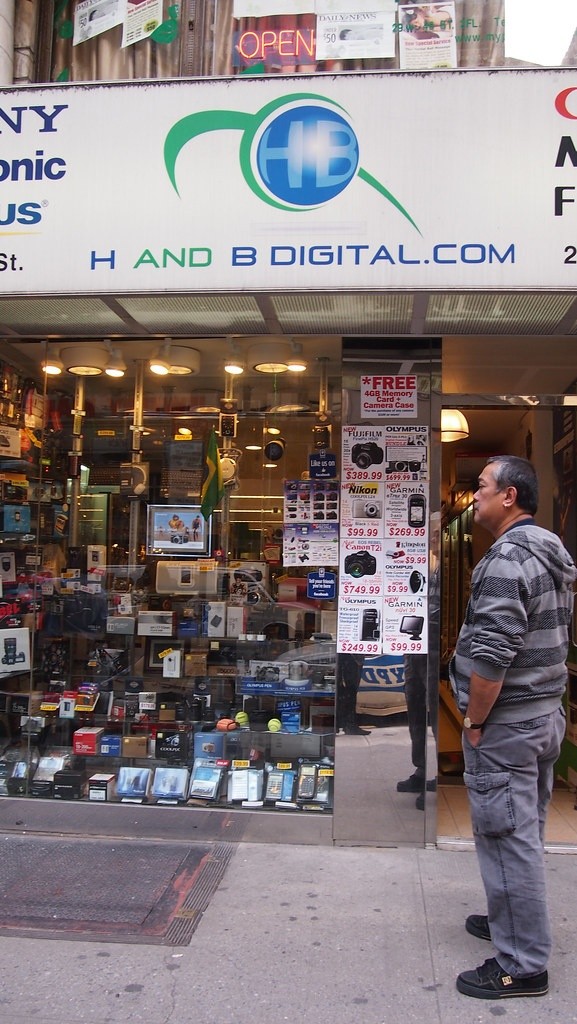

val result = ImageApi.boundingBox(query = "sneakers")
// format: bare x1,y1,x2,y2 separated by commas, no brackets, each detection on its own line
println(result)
457,957,550,1000
465,915,493,941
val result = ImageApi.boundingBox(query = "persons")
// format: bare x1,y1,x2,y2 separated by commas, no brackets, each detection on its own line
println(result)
397,511,441,809
336,654,371,735
448,457,577,1000
168,515,179,528
192,515,200,542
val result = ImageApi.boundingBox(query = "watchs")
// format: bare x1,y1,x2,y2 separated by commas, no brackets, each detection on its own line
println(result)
463,717,483,729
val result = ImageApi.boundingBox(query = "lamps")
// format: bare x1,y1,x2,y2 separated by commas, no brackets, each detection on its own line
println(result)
441,408,470,443
247,342,291,373
288,338,307,377
150,338,172,375
150,346,201,377
224,338,248,374
39,341,64,376
103,339,128,378
60,346,109,377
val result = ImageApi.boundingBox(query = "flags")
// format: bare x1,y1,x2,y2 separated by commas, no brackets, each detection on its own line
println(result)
200,428,226,521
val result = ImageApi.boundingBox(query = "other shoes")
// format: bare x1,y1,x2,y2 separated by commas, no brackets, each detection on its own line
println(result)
415,791,425,811
343,724,371,735
396,774,425,792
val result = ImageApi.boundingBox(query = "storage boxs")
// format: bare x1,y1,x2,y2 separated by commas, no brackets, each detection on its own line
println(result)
0,544,338,810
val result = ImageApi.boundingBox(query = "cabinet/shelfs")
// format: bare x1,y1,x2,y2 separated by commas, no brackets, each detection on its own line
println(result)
0,360,341,814
